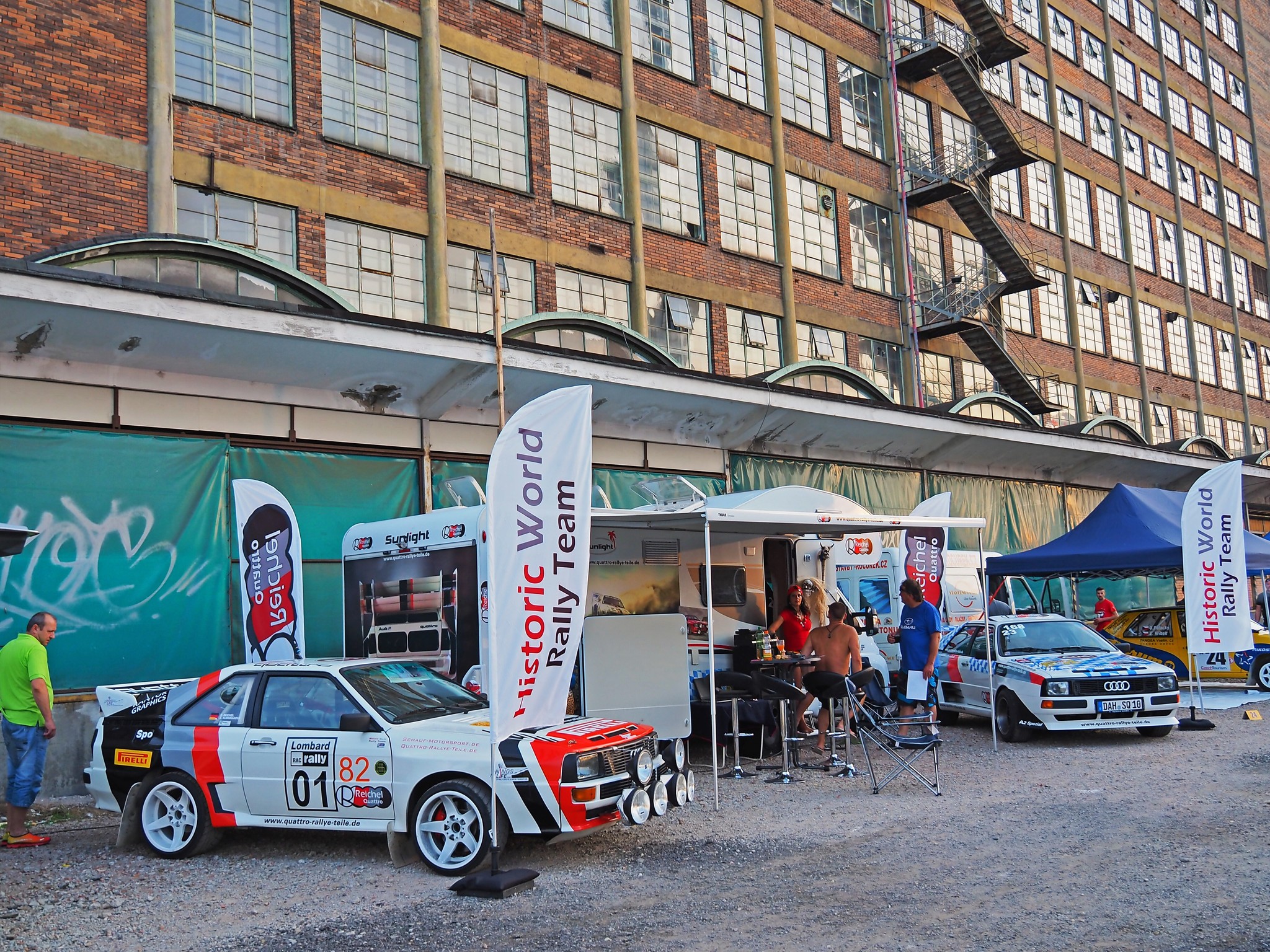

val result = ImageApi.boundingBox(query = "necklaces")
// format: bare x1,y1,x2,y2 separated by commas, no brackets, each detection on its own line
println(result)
826,624,842,639
797,609,802,620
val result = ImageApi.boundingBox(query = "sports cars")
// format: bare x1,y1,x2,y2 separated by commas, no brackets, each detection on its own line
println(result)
936,613,1180,743
81,657,696,878
1099,606,1270,693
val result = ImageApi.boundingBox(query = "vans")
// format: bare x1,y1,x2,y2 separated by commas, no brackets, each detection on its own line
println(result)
835,548,1061,686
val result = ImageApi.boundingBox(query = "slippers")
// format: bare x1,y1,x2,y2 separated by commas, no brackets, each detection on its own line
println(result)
835,728,857,739
835,744,845,749
810,744,831,750
813,746,823,756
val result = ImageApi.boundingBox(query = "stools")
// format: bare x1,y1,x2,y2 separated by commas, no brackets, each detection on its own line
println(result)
693,667,876,776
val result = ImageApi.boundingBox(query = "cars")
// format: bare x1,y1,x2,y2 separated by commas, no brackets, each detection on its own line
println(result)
591,593,631,616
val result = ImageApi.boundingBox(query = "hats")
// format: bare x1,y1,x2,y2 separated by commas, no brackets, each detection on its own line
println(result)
787,585,803,595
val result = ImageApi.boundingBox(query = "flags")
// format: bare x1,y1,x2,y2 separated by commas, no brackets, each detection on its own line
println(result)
1181,460,1256,655
899,492,949,617
231,478,306,664
485,383,593,749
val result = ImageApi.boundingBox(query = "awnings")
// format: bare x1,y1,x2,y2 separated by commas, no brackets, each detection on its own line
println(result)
593,485,986,533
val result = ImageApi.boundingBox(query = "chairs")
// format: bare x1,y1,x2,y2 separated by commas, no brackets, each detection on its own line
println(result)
845,676,942,796
851,656,899,739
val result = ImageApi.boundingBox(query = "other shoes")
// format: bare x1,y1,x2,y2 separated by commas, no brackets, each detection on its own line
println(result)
0,831,50,848
879,740,904,749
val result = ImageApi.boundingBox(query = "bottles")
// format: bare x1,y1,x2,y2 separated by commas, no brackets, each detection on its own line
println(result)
762,631,772,661
755,626,764,660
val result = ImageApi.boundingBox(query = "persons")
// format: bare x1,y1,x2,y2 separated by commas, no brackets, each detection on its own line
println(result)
1053,602,1057,612
0,612,57,848
1092,588,1120,633
767,585,815,733
1176,586,1185,606
799,602,866,756
989,589,1012,616
1255,578,1270,627
879,578,941,751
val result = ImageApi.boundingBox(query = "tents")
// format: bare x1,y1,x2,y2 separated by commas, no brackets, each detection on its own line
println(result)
985,480,1270,714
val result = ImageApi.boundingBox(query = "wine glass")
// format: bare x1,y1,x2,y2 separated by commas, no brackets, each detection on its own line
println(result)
776,640,784,657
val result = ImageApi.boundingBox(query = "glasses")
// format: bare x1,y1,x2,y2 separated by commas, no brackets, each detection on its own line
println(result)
1265,579,1270,582
899,587,911,595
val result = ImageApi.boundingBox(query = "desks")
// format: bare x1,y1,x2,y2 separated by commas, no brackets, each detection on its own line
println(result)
751,658,821,784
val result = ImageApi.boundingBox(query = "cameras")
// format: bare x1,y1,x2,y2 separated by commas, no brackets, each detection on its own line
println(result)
890,634,902,644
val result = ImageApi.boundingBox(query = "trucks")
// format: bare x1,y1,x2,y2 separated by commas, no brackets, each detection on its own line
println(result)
342,486,999,813
